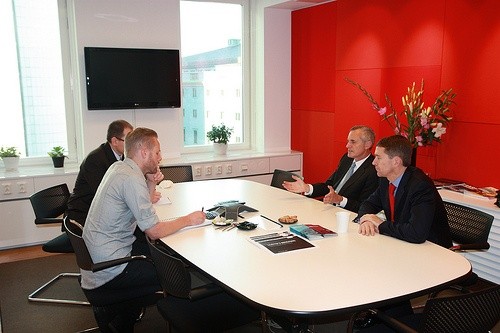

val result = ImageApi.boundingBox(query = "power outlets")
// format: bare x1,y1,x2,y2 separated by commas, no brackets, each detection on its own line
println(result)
195,162,249,177
2,183,12,196
16,182,27,193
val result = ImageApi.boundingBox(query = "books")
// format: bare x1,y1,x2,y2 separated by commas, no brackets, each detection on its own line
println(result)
290,224,338,240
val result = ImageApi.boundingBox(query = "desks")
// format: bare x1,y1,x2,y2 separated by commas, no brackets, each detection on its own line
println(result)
143,177,473,333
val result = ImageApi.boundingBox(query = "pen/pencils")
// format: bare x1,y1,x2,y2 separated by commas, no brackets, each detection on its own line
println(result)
202,207,204,212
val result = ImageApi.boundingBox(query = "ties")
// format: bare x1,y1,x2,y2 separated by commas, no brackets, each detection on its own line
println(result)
335,162,356,194
388,183,396,222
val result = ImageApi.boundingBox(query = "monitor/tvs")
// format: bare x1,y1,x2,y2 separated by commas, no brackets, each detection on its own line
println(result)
83,47,181,110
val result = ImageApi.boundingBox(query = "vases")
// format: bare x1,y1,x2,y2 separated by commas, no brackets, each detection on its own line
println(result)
412,147,417,166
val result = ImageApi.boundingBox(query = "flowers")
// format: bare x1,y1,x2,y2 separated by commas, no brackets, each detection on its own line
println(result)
344,74,459,147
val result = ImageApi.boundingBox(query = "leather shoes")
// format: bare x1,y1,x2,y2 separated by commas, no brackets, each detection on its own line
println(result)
354,314,380,328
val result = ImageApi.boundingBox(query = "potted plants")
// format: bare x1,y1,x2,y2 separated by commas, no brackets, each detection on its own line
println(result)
0,145,21,171
46,146,68,167
206,122,234,155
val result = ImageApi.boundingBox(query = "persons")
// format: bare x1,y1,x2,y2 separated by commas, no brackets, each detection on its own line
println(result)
81,128,206,333
62,120,162,232
282,126,379,212
351,134,453,333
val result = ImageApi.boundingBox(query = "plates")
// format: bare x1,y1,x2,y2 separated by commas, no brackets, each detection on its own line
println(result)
211,223,231,226
278,218,298,224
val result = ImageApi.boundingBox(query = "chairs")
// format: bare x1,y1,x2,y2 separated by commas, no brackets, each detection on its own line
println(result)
25,184,92,308
411,200,494,310
142,232,263,333
271,167,305,192
344,283,500,333
159,165,193,183
61,212,158,333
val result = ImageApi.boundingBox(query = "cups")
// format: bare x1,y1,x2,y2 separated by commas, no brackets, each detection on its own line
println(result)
336,211,351,233
